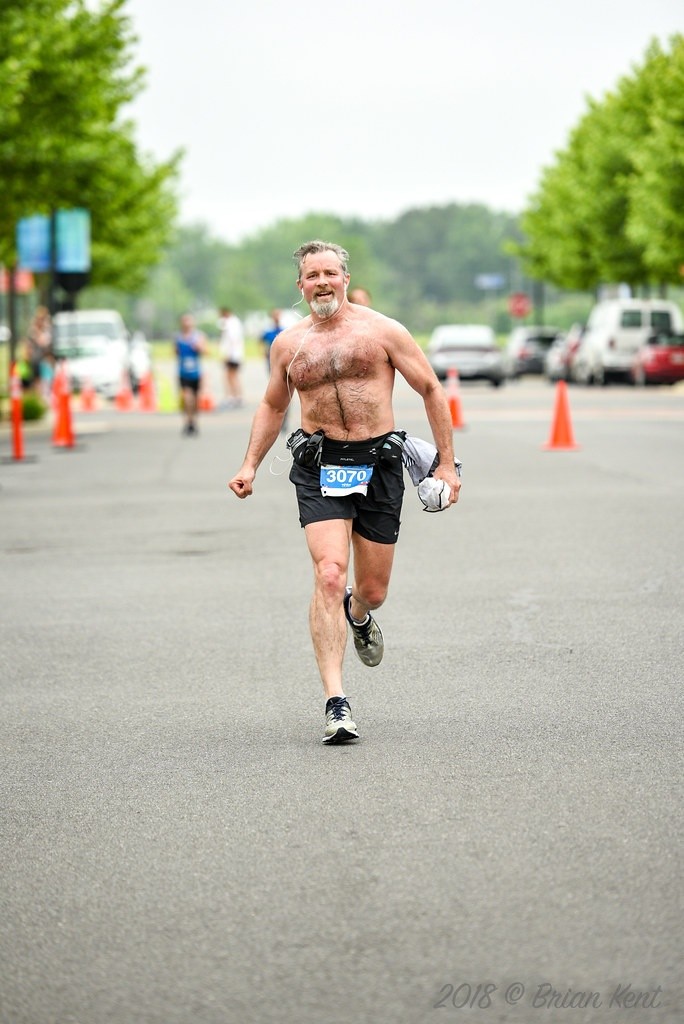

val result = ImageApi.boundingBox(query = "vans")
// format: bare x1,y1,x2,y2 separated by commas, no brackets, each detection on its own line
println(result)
575,299,684,385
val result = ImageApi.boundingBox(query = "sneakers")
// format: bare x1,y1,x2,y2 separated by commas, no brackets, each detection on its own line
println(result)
322,697,359,742
344,586,384,667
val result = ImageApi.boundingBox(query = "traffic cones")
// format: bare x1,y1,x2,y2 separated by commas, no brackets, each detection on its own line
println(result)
49,370,220,417
540,380,585,451
445,368,466,434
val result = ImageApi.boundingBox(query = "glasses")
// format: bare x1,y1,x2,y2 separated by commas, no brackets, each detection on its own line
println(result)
303,430,324,468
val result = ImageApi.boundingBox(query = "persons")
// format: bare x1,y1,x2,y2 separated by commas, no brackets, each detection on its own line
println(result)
220,307,245,400
20,307,52,388
260,311,292,433
174,312,205,435
230,242,461,744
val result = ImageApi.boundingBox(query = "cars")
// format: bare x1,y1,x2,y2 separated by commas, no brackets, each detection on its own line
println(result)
544,321,584,384
629,330,684,388
52,309,151,396
503,325,559,380
424,323,507,388
